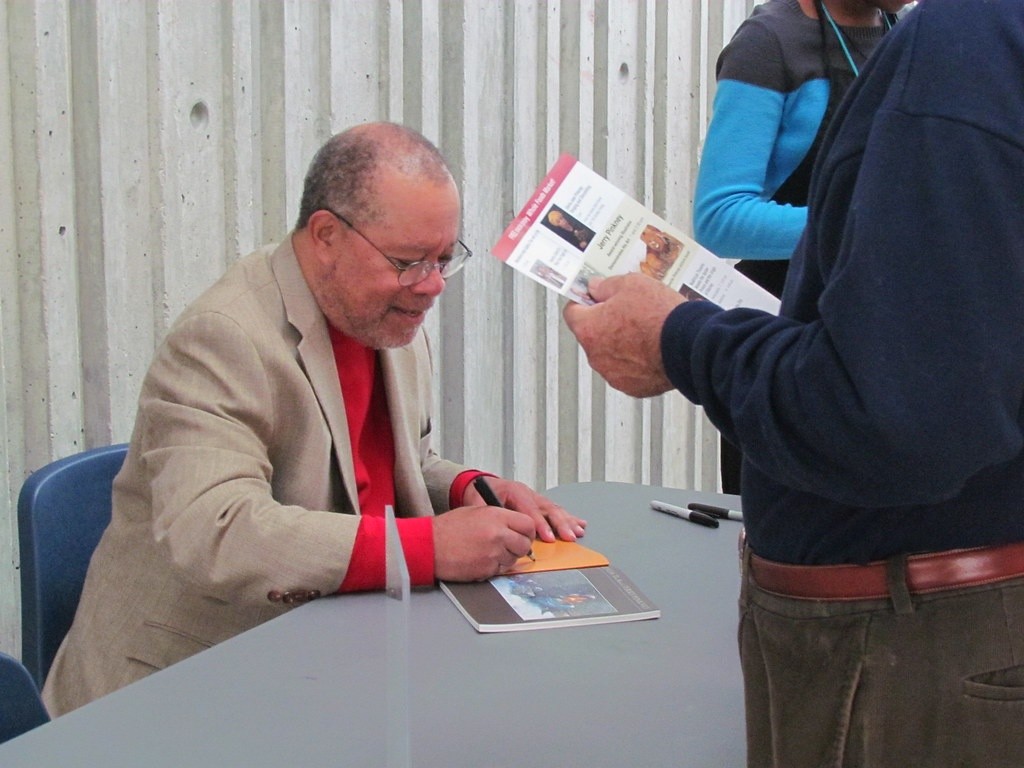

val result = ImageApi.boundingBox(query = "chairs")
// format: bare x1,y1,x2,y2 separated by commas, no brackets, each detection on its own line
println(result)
0,445,135,744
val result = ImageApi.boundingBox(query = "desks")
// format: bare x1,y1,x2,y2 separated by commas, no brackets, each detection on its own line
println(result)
0,479,753,767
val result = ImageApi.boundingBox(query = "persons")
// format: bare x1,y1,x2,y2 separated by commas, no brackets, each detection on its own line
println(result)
696,0,929,493
547,209,594,250
561,0,1024,768
41,124,587,722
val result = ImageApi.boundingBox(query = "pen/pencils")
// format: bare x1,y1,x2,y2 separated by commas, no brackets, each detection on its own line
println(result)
472,476,536,563
650,499,720,530
687,503,743,522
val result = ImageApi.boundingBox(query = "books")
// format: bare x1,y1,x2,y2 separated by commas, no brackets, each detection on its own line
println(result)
438,534,659,632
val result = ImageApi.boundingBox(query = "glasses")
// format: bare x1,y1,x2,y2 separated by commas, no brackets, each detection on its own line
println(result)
317,206,473,286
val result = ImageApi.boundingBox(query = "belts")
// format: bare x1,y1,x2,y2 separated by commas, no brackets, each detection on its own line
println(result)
738,528,1024,601
